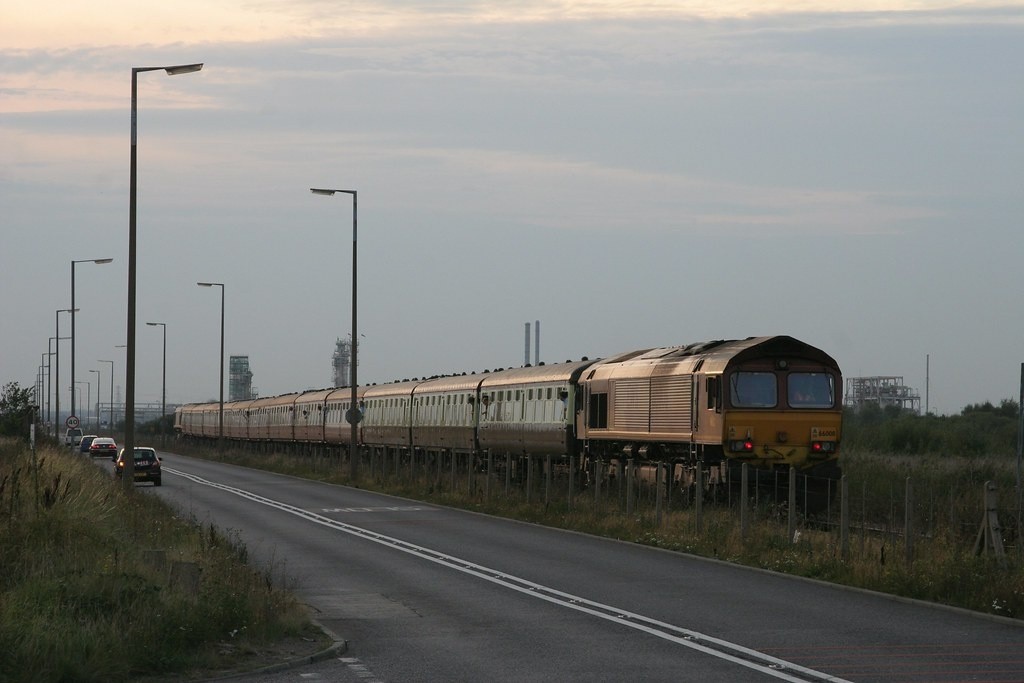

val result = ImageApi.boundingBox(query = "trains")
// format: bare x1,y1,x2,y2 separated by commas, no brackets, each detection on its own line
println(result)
168,333,846,520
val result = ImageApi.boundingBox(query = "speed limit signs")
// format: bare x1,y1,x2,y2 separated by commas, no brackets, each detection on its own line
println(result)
66,416,79,429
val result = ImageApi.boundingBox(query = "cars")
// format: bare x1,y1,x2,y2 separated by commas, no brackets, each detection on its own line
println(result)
88,436,118,459
79,434,99,452
111,445,163,486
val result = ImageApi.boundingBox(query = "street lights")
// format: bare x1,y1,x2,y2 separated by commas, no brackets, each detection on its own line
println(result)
67,344,128,438
146,322,167,449
69,258,115,450
54,309,81,446
121,61,206,485
309,187,363,484
32,352,57,425
48,337,72,441
197,282,225,454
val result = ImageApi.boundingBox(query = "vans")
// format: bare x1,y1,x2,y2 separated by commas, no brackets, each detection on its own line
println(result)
64,427,83,446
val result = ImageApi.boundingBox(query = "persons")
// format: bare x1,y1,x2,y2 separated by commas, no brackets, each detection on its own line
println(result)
792,379,816,401
